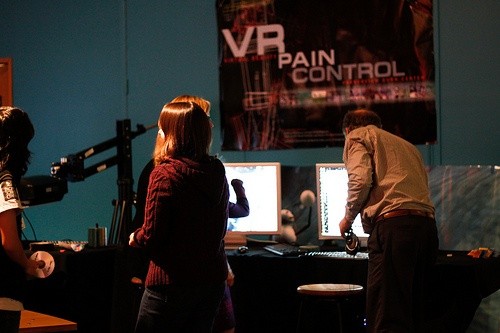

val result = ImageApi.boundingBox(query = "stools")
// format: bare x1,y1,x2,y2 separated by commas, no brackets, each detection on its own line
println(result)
296,283,363,333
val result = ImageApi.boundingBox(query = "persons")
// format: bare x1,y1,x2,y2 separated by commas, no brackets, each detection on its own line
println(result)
129,102,228,333
0,105,43,275
228,179,249,218
155,96,236,333
339,110,439,333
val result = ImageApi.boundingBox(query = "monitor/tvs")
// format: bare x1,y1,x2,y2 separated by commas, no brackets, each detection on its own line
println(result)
315,163,370,251
224,163,282,250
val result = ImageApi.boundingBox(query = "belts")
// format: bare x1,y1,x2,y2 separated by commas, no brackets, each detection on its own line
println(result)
375,209,435,224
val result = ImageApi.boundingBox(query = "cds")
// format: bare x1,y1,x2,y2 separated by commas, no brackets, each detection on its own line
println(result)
31,251,56,278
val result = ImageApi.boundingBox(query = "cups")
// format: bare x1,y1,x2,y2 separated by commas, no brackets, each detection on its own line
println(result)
98,228,108,246
89,228,98,248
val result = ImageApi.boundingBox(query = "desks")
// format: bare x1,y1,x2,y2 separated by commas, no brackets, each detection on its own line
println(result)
224,244,500,333
19,239,117,333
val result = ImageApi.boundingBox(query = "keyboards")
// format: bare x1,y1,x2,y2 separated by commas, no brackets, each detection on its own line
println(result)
305,251,370,260
263,244,302,257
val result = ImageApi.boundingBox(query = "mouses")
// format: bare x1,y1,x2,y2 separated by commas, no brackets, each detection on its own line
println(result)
238,246,248,253
